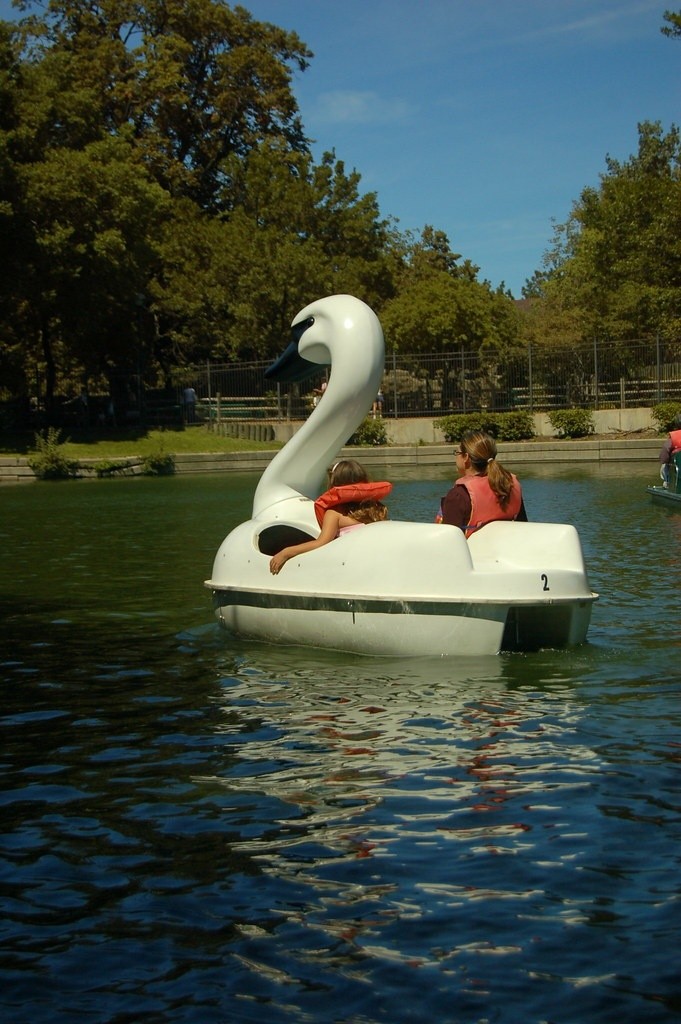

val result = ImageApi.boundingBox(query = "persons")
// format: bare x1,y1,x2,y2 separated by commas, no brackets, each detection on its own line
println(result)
270,460,388,575
185,382,199,424
310,376,384,421
660,414,681,489
436,432,529,539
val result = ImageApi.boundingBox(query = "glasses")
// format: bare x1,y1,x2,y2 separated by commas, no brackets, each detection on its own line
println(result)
452,450,464,456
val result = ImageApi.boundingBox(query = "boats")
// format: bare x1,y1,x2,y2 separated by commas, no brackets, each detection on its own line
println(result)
204,294,600,658
643,450,681,502
